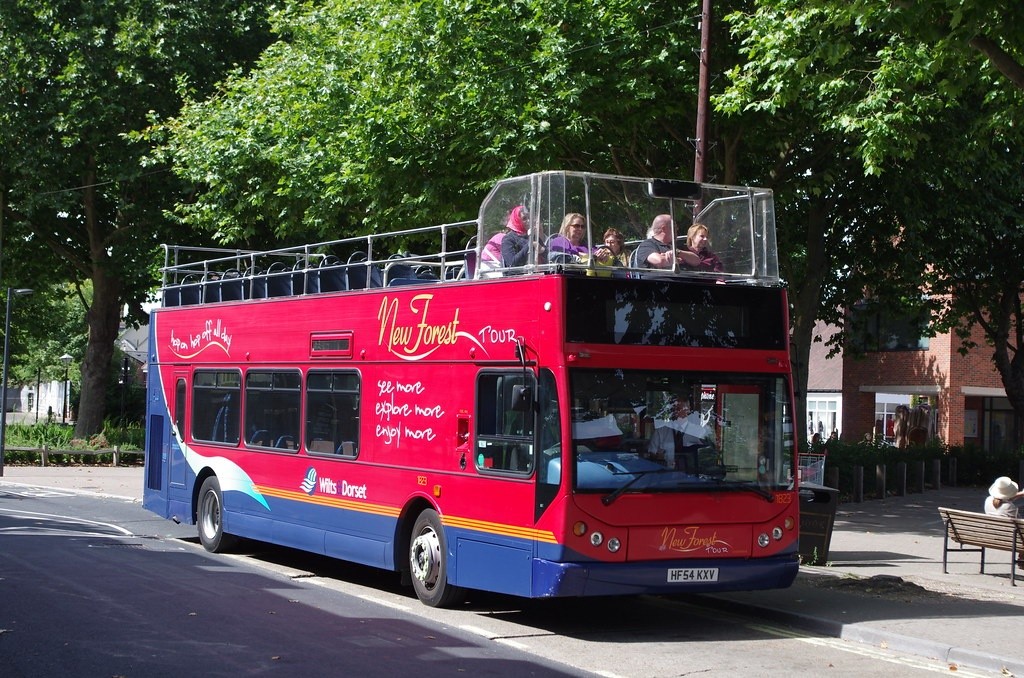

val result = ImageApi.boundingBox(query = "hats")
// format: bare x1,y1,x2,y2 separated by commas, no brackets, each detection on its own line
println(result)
988,476,1019,500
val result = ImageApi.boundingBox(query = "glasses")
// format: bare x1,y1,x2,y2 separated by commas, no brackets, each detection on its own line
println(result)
570,224,585,229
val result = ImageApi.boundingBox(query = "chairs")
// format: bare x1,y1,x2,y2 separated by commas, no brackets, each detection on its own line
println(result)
166,228,638,472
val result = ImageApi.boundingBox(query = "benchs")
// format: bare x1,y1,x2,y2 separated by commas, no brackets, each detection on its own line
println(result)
937,507,1024,586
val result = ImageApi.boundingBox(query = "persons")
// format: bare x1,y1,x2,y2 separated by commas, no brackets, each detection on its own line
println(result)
810,431,837,449
481,207,724,281
864,433,872,446
984,476,1024,570
646,398,712,470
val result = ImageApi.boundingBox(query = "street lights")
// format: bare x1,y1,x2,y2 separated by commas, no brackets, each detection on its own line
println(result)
60,352,74,426
0,287,35,477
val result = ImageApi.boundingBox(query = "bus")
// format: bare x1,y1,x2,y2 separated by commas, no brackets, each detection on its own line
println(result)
141,170,804,610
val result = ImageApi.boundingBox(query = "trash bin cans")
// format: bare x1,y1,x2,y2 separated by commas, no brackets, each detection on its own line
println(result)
796,481,839,567
